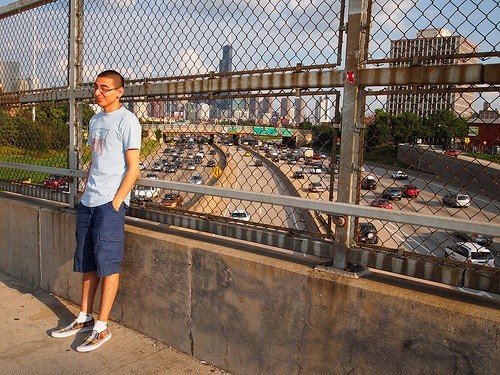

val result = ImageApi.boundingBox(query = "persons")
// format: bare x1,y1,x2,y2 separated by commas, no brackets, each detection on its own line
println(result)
50,70,142,352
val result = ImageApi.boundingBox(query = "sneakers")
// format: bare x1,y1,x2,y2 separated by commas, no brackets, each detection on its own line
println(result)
51,317,94,338
75,328,112,352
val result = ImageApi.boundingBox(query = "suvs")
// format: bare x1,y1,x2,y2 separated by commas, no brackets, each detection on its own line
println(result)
382,187,401,200
357,222,379,245
370,199,393,209
399,185,419,198
442,192,470,208
445,242,495,268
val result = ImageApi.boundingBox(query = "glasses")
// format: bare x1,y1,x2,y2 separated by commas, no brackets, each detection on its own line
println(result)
92,85,122,94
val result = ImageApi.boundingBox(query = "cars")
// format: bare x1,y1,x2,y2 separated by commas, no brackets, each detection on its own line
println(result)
13,134,217,208
444,150,460,156
455,231,494,244
235,146,242,150
256,159,263,166
244,138,408,194
245,151,251,156
228,142,233,145
228,210,250,221
